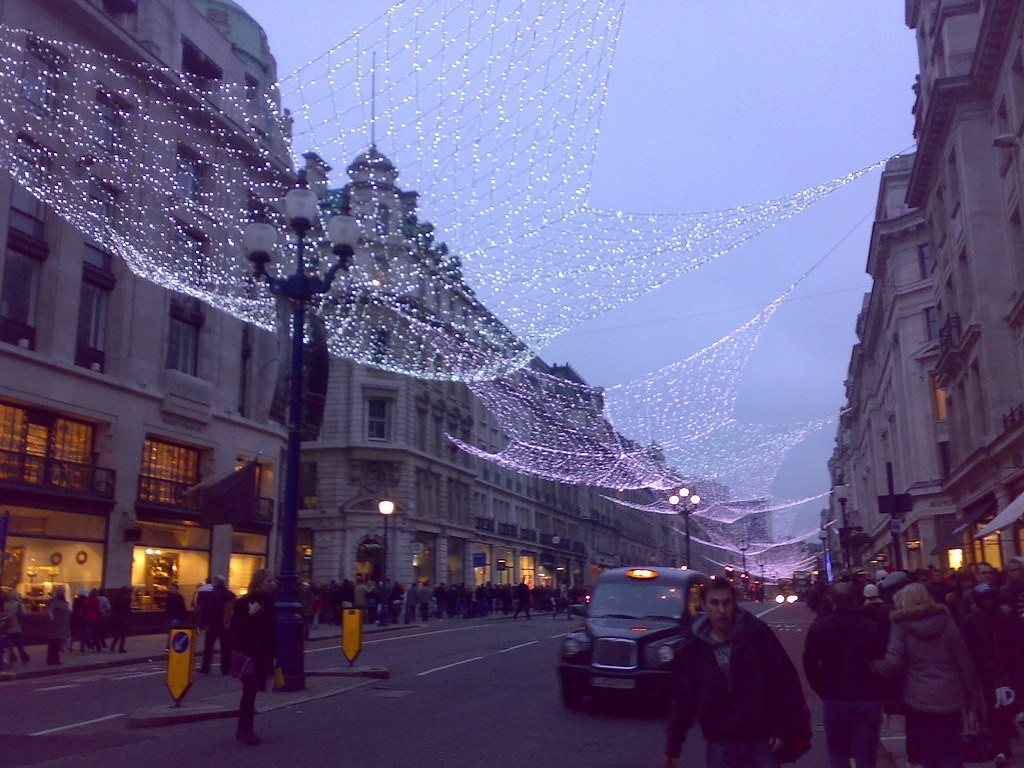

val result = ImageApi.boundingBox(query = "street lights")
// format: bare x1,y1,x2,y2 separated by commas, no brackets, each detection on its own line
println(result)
819,527,828,582
758,557,765,603
834,473,851,570
242,168,361,692
735,538,748,570
378,487,394,625
669,483,700,570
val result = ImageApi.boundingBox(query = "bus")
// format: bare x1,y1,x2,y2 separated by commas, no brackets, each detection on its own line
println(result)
777,577,794,604
793,571,811,602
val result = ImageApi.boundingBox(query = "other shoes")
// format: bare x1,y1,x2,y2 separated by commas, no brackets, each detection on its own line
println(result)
195,667,210,676
993,753,1007,768
235,729,262,746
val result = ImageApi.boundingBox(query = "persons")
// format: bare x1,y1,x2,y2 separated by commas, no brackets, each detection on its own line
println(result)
0,584,134,665
801,581,884,768
232,567,277,746
661,576,807,767
299,573,592,629
165,574,237,674
809,558,1024,768
870,581,974,768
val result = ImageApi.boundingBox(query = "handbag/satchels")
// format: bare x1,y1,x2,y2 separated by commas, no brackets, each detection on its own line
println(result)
263,656,285,694
223,595,238,631
763,700,815,765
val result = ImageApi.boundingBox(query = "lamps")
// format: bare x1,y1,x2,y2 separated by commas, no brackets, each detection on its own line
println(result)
992,132,1019,153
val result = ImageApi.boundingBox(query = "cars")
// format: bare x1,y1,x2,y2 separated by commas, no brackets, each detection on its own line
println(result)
557,566,710,711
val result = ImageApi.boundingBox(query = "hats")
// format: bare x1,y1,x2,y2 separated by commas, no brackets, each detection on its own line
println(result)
974,582,992,599
829,581,856,600
850,566,864,575
958,570,976,582
875,569,889,580
863,584,880,598
1008,557,1024,566
838,568,849,577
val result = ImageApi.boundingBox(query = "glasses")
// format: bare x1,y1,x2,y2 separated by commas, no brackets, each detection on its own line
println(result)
980,570,992,576
